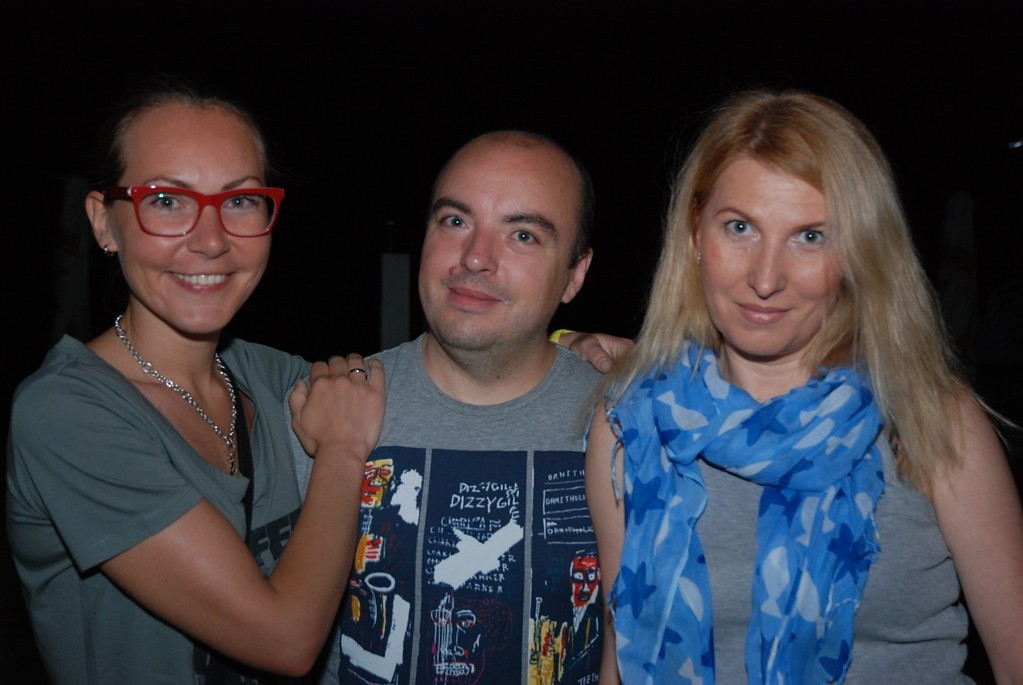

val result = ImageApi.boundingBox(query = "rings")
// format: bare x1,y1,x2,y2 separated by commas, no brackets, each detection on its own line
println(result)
349,368,368,381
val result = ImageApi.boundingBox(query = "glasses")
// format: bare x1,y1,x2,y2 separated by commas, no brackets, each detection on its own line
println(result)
103,185,285,238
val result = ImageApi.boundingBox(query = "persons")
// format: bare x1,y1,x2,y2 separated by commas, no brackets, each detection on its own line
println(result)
282,131,605,685
584,94,1023,685
5,78,635,685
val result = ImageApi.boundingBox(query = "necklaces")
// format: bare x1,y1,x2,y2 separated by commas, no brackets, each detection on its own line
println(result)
115,315,237,477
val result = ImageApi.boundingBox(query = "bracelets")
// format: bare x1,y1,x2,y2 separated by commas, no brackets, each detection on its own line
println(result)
550,330,574,343
